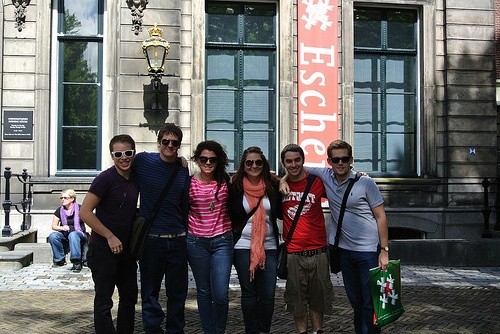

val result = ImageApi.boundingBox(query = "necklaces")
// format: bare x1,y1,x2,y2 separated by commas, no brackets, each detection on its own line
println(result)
197,173,217,212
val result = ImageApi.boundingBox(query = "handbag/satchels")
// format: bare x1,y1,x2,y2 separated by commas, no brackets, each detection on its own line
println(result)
126,213,149,259
369,258,405,327
277,243,287,278
329,243,352,274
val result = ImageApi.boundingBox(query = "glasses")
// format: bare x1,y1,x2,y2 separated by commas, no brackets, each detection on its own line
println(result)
245,159,262,166
331,157,351,163
60,197,72,200
161,139,181,147
111,149,134,158
198,156,218,164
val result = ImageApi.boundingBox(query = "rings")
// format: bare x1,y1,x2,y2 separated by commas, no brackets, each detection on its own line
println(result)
114,250,117,252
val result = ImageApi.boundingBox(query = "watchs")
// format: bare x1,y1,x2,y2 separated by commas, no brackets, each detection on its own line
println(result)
381,246,389,252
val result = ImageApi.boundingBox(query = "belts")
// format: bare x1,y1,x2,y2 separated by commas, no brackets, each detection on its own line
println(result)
289,246,327,256
149,232,186,239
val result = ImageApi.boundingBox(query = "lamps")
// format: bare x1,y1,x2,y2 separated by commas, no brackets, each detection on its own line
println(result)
141,22,169,90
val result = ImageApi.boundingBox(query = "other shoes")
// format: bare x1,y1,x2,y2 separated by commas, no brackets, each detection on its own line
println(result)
58,257,66,266
73,262,81,270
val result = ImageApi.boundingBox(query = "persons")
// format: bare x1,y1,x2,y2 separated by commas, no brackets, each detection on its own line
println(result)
48,190,85,272
279,143,369,334
229,147,281,334
278,138,389,334
79,134,188,334
132,123,190,334
187,141,232,334
